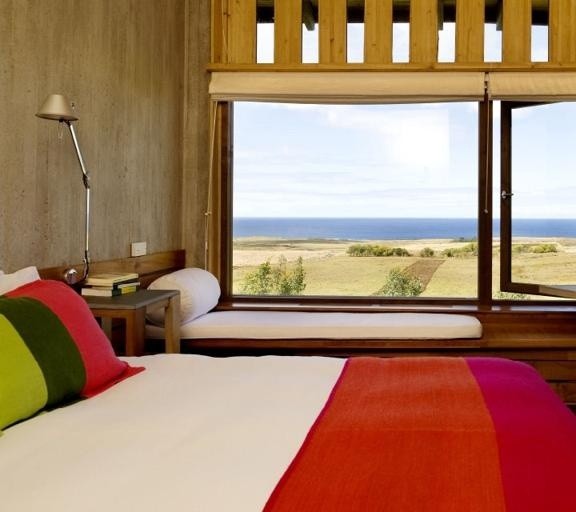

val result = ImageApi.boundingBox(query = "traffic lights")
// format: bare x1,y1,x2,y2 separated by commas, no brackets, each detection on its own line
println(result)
144,268,225,327
2,278,146,439
1,264,40,297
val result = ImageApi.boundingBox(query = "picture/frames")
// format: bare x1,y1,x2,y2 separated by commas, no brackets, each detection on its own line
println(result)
84,289,182,358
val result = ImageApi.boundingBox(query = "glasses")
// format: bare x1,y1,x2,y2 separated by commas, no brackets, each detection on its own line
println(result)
33,93,96,294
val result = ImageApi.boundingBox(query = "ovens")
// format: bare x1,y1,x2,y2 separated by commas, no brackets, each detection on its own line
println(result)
1,267,574,511
136,266,574,352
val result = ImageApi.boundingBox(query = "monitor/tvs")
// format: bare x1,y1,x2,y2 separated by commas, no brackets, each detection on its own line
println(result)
81,272,140,297
